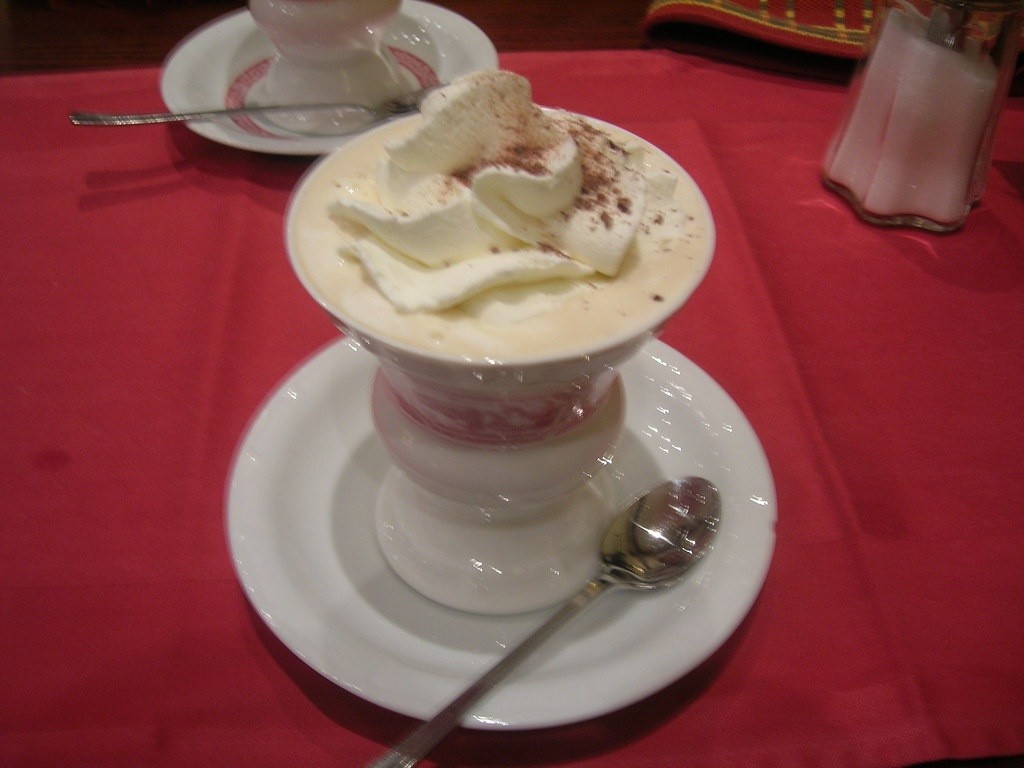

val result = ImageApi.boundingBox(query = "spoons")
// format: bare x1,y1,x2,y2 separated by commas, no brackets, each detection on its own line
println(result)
359,477,722,768
68,86,449,126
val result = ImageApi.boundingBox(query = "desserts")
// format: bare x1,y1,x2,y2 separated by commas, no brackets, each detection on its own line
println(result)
287,66,707,360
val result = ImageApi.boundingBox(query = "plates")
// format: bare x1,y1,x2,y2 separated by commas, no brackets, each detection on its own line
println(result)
222,333,777,731
158,1,499,154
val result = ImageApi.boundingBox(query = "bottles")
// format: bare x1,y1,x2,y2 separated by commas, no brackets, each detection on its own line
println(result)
817,0,1024,231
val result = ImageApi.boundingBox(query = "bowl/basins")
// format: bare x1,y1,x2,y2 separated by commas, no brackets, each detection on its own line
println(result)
282,110,716,618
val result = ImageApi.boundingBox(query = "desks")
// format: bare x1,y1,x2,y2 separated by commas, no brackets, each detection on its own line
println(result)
0,51,1024,768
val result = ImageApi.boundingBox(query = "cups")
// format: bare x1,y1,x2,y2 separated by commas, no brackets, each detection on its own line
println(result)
247,0,403,136
